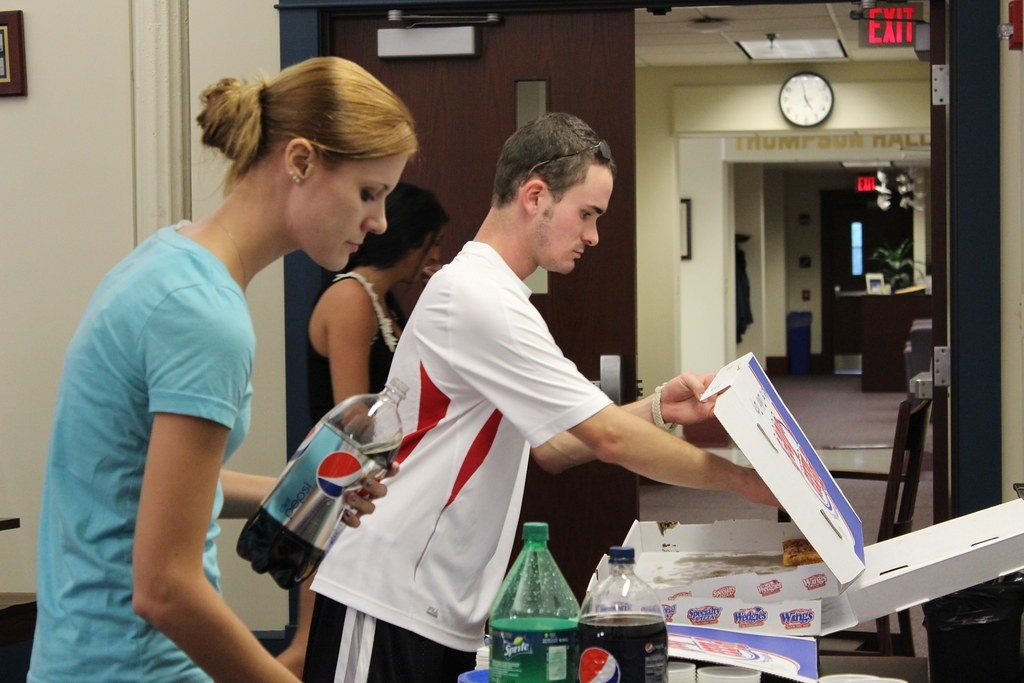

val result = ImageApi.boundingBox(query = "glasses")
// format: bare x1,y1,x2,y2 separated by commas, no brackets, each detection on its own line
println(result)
527,139,611,180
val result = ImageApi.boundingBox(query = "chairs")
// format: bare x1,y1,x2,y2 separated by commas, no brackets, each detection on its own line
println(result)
817,398,930,657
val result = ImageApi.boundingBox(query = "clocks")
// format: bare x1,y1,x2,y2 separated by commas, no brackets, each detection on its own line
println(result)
778,71,834,127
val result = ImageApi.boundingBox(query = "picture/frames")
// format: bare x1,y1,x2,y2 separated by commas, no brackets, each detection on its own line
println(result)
680,199,692,259
0,9,28,98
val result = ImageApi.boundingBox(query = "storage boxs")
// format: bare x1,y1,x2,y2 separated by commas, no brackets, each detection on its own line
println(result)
582,352,1024,682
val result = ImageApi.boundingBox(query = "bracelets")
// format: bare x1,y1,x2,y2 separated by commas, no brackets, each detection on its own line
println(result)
652,381,679,434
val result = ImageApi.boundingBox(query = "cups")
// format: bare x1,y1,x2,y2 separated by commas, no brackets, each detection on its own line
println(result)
668,662,761,683
458,670,490,683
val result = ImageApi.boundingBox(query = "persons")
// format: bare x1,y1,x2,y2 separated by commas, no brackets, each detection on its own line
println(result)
25,58,418,683
272,183,446,683
304,112,786,683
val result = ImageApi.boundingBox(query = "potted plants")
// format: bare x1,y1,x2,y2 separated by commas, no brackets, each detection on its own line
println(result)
871,239,926,290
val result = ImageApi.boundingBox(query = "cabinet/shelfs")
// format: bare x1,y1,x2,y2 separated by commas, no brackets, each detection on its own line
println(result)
861,295,930,392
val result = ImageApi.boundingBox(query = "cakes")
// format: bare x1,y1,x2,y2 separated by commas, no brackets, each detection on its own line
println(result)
782,539,823,565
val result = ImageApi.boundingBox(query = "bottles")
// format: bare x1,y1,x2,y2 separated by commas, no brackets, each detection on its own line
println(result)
237,377,410,590
488,523,580,683
577,547,668,683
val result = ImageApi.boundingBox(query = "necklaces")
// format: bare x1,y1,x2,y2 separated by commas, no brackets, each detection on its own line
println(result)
213,218,245,287
